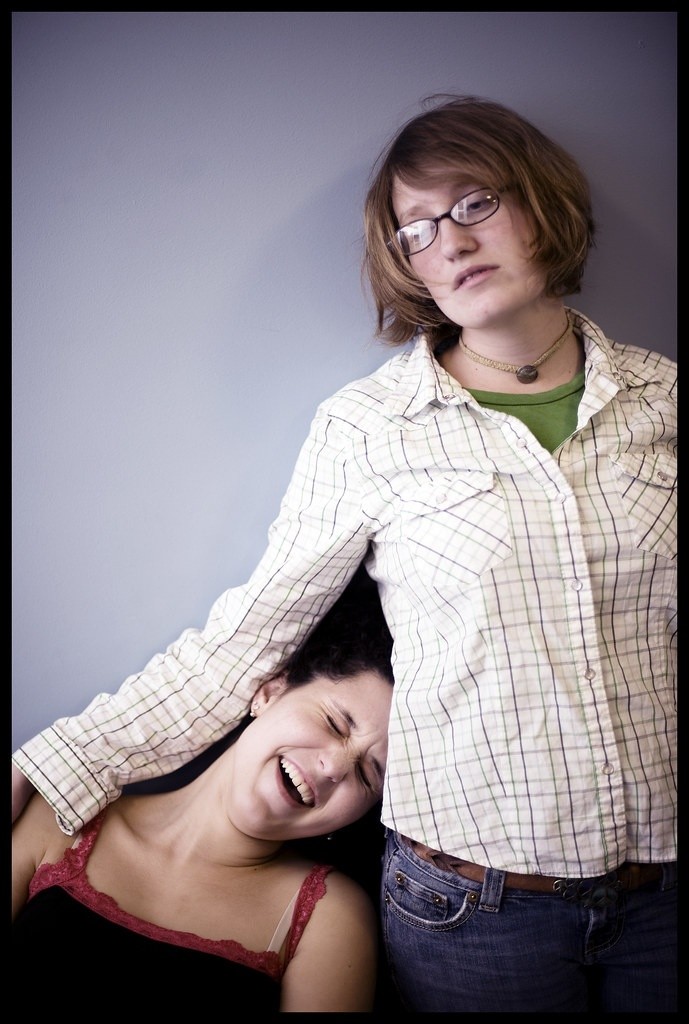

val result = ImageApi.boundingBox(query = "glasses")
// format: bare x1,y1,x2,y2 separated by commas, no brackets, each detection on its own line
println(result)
386,183,520,256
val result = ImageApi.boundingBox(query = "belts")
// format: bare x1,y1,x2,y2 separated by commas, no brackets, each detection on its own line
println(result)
400,835,662,908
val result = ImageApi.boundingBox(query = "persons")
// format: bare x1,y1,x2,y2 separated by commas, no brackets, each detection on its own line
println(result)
10,91,678,1011
13,585,395,1012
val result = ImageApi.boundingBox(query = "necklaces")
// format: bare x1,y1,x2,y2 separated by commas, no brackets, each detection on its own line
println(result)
457,312,575,387
